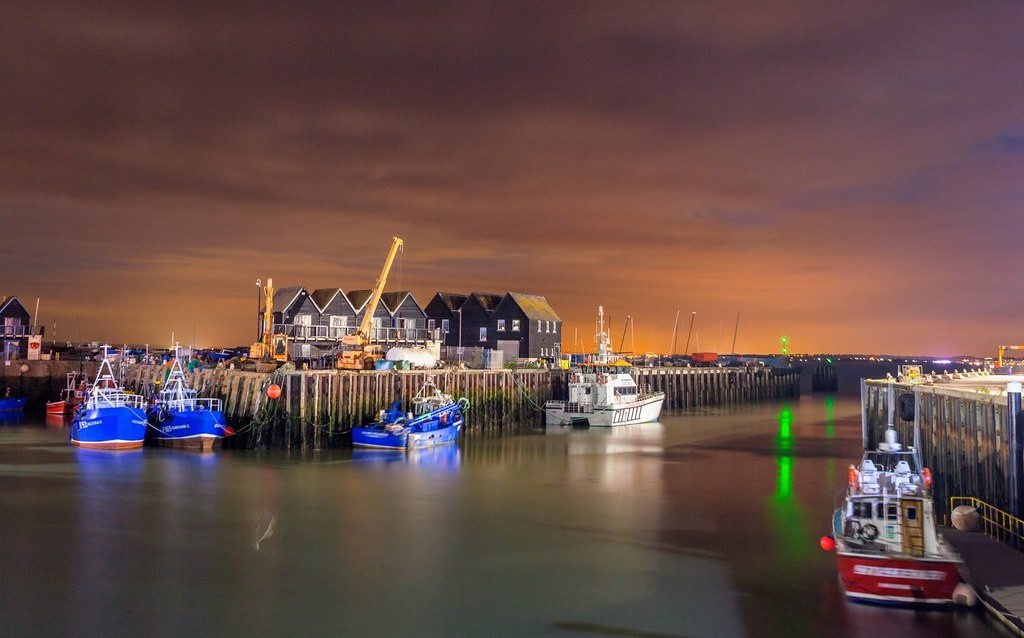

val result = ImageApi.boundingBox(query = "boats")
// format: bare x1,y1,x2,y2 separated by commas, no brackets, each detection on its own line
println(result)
351,443,462,485
68,345,148,449
143,342,229,451
45,400,70,414
543,306,663,426
817,431,977,606
0,411,26,425
45,413,67,425
348,370,470,450
0,386,26,411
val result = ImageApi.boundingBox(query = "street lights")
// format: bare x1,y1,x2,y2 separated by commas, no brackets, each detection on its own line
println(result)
450,308,461,367
255,279,261,343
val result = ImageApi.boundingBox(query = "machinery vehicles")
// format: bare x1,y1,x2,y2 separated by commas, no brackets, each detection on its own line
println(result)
334,233,406,371
244,278,288,371
